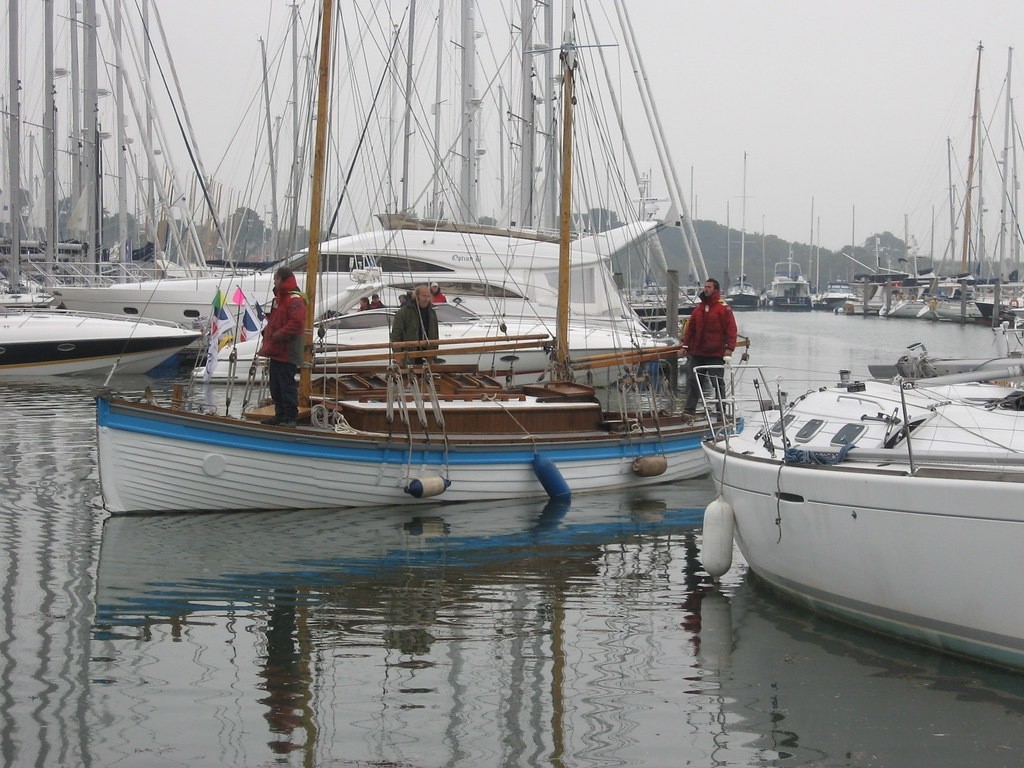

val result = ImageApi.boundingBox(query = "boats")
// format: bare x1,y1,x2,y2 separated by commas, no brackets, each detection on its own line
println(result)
821,280,856,308
692,321,1024,672
767,245,812,312
0,309,201,377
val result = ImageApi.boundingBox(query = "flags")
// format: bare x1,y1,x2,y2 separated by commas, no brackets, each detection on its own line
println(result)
203,288,268,384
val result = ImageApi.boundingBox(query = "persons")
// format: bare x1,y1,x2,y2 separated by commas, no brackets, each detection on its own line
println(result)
358,294,385,312
675,278,737,428
389,285,439,384
398,282,446,308
257,268,306,429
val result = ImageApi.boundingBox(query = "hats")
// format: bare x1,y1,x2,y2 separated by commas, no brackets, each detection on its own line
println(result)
372,294,378,300
431,282,438,287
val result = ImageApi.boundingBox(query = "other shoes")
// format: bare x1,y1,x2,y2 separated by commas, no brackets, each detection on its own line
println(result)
260,414,280,425
717,412,726,422
277,420,296,428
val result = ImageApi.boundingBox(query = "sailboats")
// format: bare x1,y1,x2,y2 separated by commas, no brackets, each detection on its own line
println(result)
93,476,716,768
19,1,705,384
725,151,761,311
92,1,750,514
844,40,1024,322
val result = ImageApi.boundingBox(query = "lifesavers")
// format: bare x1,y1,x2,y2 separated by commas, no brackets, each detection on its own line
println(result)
218,335,233,350
